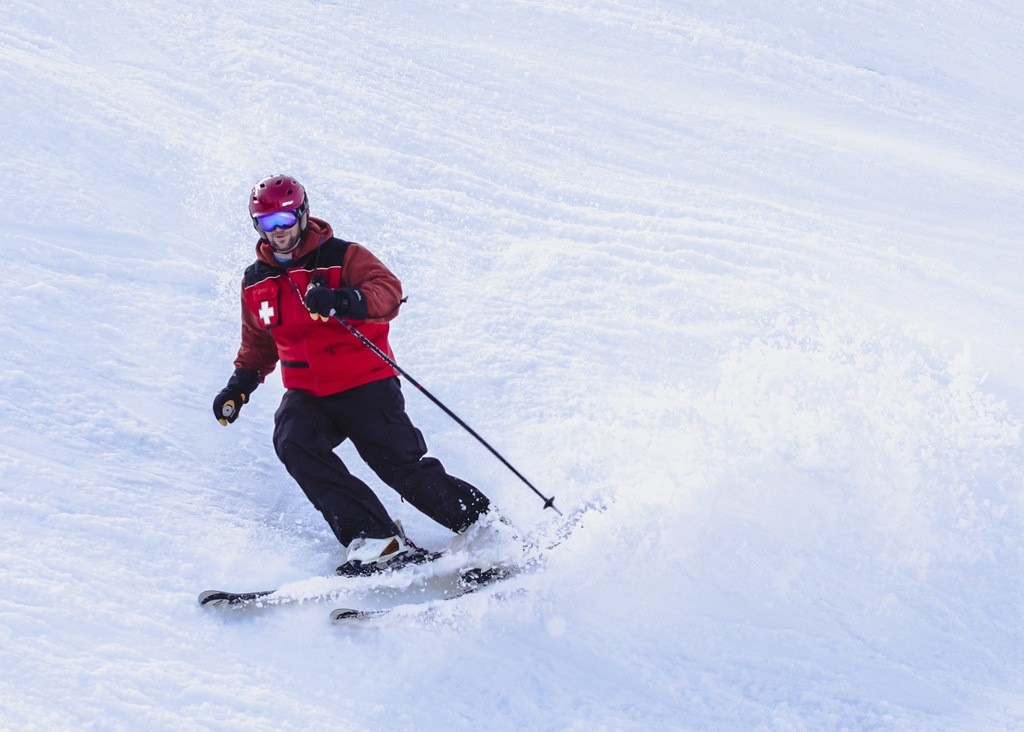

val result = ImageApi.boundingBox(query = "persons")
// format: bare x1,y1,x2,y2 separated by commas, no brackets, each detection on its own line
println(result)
213,174,511,576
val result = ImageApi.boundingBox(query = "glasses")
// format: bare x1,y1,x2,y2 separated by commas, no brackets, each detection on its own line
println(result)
255,209,301,232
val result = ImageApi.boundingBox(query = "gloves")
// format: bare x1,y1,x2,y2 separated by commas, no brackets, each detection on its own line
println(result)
212,366,261,427
305,287,369,323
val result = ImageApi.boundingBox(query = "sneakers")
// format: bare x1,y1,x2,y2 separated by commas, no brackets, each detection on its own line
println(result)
346,533,412,564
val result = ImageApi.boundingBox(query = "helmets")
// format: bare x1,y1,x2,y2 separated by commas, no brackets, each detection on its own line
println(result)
248,173,310,219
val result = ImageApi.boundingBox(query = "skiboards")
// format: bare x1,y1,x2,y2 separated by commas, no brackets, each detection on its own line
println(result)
196,493,609,628
